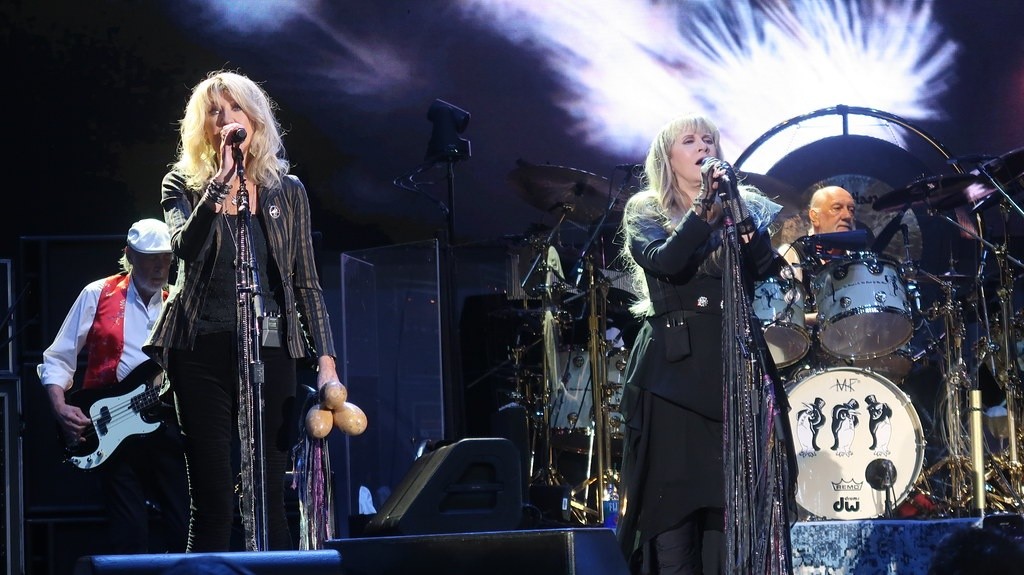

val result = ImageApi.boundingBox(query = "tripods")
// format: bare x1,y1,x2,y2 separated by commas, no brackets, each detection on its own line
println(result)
519,170,633,526
908,159,1024,514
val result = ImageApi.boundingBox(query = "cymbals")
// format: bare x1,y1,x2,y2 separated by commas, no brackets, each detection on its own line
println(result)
909,271,976,285
711,171,798,223
503,164,632,226
872,145,1024,213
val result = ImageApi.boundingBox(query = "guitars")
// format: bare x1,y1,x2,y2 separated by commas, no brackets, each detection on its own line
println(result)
54,358,173,474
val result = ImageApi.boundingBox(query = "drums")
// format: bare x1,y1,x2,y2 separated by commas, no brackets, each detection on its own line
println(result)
751,277,811,369
547,339,632,460
834,337,914,386
812,251,915,362
783,365,927,521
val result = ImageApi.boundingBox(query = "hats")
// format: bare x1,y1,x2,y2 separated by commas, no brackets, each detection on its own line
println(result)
126,219,173,254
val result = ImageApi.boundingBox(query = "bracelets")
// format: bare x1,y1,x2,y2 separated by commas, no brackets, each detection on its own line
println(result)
204,178,233,204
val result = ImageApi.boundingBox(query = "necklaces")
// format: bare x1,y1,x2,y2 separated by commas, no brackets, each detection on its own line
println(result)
223,181,255,267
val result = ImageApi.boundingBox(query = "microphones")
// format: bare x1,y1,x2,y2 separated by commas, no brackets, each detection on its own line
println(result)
616,163,645,172
230,127,247,142
958,154,997,160
702,157,730,184
879,460,893,475
870,203,911,254
805,228,868,251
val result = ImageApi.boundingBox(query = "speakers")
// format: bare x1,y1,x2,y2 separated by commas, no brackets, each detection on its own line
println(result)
322,526,631,575
362,436,524,538
73,549,342,575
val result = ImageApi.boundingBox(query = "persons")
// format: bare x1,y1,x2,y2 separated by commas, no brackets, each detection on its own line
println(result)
777,186,856,323
606,112,799,575
142,62,340,552
37,218,198,554
